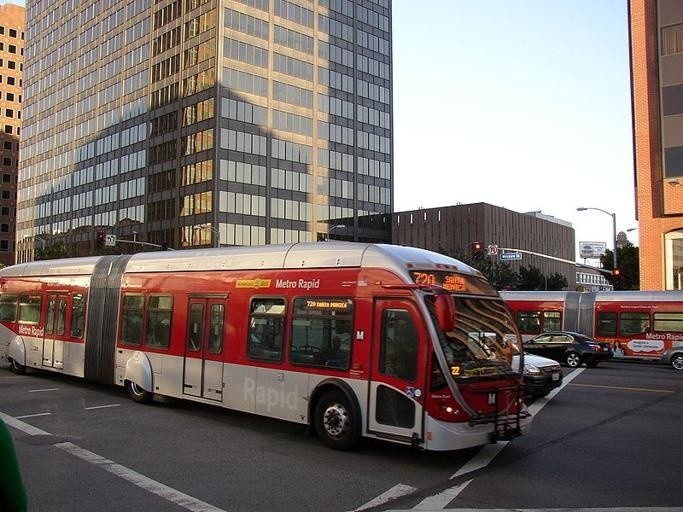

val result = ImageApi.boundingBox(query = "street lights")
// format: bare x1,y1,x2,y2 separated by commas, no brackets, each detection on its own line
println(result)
326,225,346,239
577,207,617,269
627,228,640,232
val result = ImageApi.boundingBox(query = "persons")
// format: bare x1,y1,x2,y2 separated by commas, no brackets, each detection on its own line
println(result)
611,340,625,357
324,335,345,367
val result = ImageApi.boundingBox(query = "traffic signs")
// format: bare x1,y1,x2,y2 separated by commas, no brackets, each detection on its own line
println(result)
500,253,522,261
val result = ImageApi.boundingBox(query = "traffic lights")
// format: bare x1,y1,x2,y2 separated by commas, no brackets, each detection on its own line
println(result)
97,229,105,249
471,241,485,260
613,268,621,291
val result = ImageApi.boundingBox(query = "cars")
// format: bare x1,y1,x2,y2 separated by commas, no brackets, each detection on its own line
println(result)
511,343,563,399
662,345,683,370
523,331,614,368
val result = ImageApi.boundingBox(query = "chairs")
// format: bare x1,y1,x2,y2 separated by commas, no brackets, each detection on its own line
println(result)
249,343,347,369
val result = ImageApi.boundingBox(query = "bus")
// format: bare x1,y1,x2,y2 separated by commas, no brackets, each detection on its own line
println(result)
0,240,533,452
498,289,683,364
